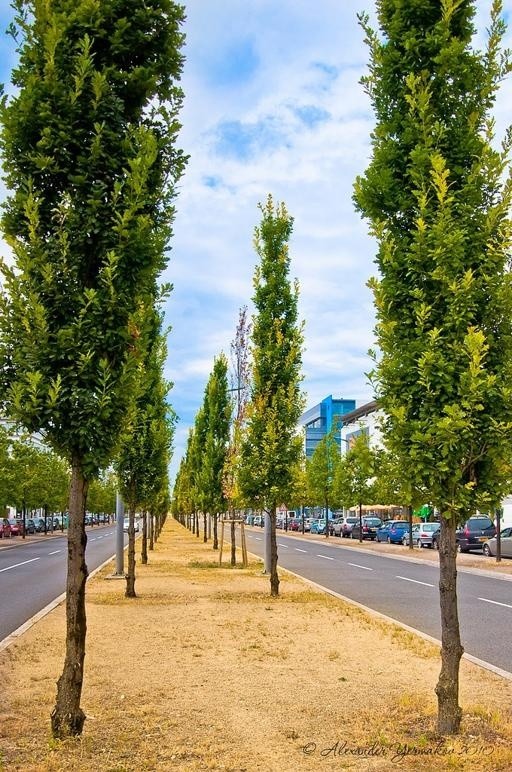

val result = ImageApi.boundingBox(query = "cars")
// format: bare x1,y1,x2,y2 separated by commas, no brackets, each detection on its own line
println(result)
242,510,512,559
121,517,139,532
0,512,109,540
